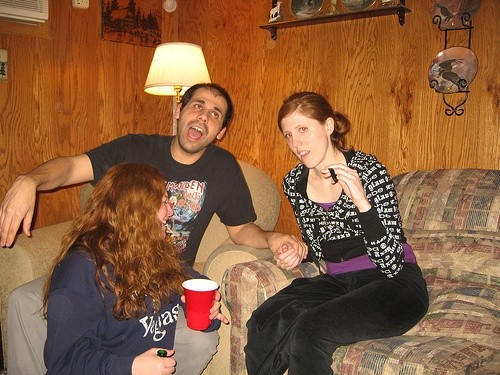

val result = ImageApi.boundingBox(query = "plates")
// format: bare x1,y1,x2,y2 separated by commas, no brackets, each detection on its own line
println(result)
432,0,481,28
428,46,477,93
290,0,322,19
343,0,376,12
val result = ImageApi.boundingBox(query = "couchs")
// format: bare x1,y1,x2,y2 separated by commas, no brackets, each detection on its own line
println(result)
220,169,500,375
0,160,282,375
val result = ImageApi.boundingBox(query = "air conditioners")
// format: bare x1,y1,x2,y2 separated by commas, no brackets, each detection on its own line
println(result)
0,0,49,26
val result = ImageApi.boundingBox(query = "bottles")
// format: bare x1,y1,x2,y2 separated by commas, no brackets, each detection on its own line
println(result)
157,350,167,357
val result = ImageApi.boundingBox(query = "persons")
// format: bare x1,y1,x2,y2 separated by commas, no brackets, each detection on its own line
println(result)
44,162,229,375
243,91,429,375
0,82,309,375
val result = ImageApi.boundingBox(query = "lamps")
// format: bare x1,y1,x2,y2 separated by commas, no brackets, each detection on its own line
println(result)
144,42,212,137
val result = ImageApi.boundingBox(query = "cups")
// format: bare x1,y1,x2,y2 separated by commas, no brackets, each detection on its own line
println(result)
181,278,219,331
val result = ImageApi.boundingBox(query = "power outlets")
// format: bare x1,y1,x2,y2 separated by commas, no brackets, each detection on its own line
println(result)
73,0,90,10
